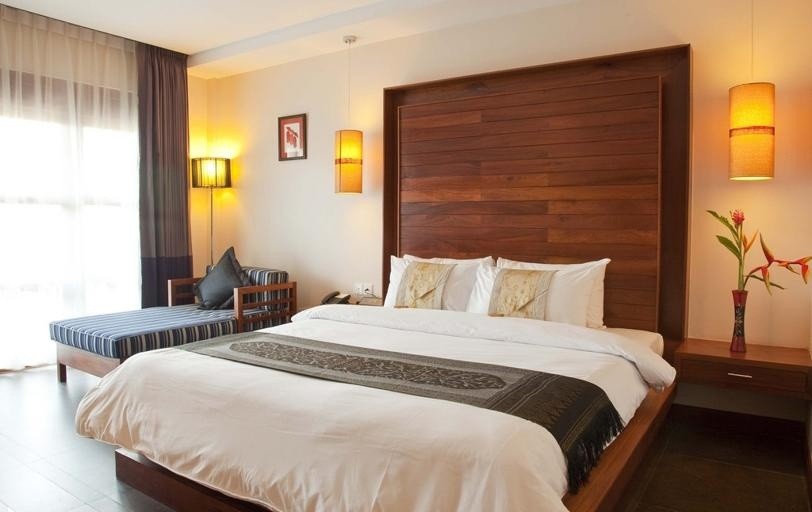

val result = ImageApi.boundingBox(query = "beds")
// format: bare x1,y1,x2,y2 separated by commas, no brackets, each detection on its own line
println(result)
49,265,297,384
73,300,678,512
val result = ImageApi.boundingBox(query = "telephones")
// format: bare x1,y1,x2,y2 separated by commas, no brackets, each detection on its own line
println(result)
320,291,351,304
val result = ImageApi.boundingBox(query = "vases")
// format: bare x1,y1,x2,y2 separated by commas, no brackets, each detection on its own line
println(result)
729,289,749,353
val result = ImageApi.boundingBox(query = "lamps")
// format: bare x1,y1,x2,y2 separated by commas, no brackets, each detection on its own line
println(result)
728,0,777,182
191,157,232,264
334,34,364,196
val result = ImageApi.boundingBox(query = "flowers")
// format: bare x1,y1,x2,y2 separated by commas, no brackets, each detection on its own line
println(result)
706,207,812,295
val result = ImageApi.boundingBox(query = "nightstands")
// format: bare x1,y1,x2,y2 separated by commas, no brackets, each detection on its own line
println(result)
676,338,811,465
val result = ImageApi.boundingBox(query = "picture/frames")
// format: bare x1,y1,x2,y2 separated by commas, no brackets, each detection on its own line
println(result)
277,113,309,161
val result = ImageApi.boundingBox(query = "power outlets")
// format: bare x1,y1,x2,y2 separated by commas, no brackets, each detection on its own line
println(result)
362,284,374,298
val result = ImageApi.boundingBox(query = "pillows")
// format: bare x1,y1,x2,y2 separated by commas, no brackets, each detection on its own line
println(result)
486,267,595,328
383,252,494,310
497,255,612,328
192,246,251,309
401,252,496,268
